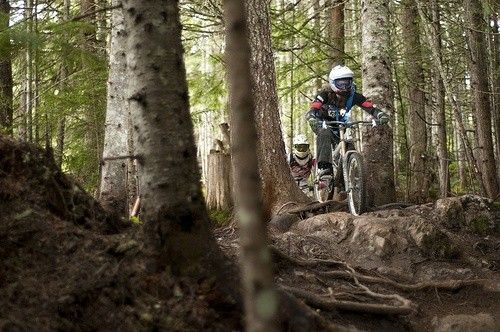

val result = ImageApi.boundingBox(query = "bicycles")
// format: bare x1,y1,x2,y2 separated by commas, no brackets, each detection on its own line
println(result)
312,118,381,216
292,167,314,198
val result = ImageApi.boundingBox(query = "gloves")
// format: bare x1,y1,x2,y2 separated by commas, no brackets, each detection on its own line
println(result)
377,113,390,125
309,116,319,127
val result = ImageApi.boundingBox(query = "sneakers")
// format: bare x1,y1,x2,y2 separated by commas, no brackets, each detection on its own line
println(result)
317,162,333,181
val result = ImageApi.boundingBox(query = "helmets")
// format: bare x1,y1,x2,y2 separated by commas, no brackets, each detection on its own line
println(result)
292,134,311,159
328,65,354,94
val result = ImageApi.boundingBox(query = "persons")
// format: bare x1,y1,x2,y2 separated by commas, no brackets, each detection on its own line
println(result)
306,65,390,195
287,134,315,198
